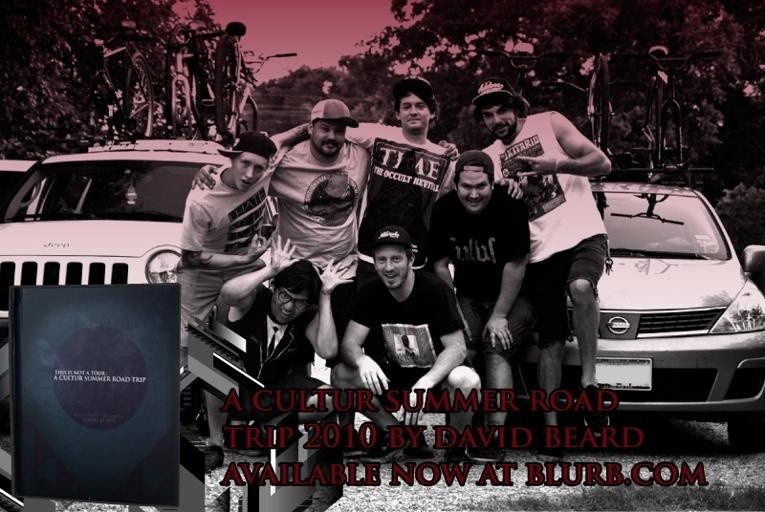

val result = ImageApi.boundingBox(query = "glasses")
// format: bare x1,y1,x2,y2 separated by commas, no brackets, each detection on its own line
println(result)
276,288,308,310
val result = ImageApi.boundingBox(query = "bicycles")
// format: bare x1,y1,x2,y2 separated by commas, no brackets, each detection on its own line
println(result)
67,0,301,147
474,34,725,186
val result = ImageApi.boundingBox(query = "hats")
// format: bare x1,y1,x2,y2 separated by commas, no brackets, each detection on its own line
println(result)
368,224,411,251
471,77,515,104
311,99,359,128
217,130,277,161
393,74,436,106
455,150,493,177
270,259,321,303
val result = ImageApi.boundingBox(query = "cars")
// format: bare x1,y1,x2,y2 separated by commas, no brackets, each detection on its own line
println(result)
510,179,765,460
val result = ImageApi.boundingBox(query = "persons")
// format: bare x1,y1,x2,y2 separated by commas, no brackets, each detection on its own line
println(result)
179,75,613,463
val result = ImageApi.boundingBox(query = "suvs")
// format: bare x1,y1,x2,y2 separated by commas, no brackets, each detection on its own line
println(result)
0,134,281,408
